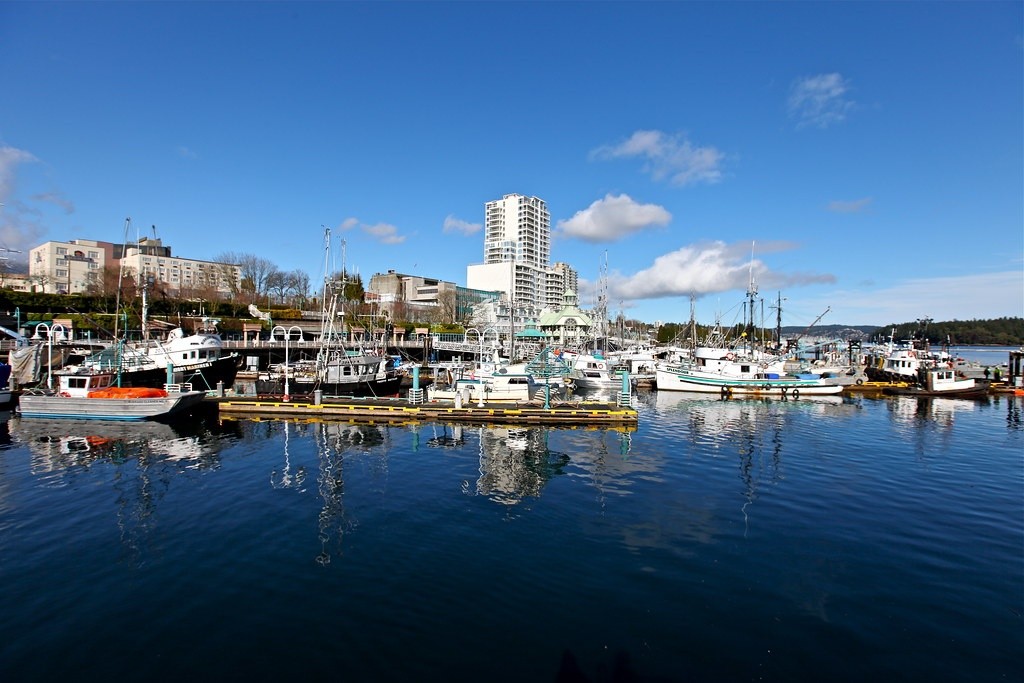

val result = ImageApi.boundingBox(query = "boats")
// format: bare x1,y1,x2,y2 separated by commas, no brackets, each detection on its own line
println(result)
428,371,560,402
256,226,405,396
85,228,242,392
547,249,700,381
886,317,953,377
18,338,204,421
882,360,991,395
654,242,843,396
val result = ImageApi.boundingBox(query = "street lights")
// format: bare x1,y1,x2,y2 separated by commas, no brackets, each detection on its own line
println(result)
461,328,503,408
266,325,305,403
29,323,67,389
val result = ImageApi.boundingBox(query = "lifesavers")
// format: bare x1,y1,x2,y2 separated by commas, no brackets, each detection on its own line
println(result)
781,386,788,392
726,352,734,360
722,385,729,393
792,389,799,394
598,363,603,370
765,383,772,390
856,379,863,385
